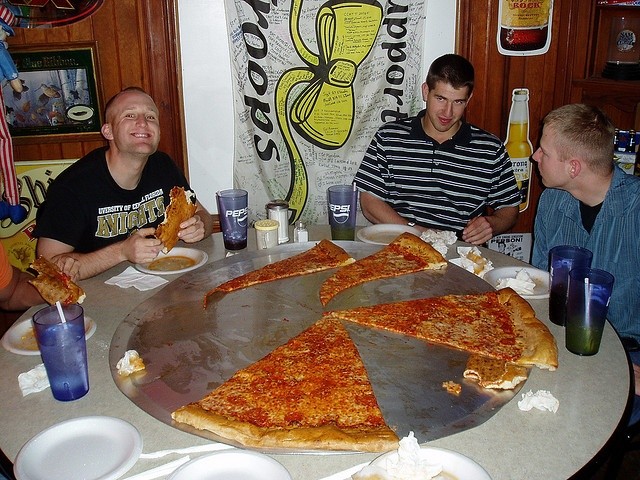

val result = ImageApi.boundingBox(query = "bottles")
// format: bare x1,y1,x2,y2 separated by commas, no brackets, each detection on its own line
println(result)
294,221,309,243
254,219,280,250
496,0,554,57
624,129,635,152
503,88,534,214
613,128,620,152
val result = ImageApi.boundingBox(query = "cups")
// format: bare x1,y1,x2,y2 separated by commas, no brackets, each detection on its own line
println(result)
548,244,593,327
565,267,615,356
215,189,248,250
33,303,90,401
266,200,297,244
328,185,358,241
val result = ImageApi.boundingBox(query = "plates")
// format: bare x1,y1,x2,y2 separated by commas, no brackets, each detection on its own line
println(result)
135,246,209,275
12,415,144,480
357,223,422,245
166,448,293,480
484,266,550,300
0,318,97,356
359,444,492,480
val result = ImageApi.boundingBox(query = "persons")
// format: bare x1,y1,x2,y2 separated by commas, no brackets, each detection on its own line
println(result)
36,86,214,280
0,5,28,225
0,242,81,312
532,103,639,425
352,53,523,246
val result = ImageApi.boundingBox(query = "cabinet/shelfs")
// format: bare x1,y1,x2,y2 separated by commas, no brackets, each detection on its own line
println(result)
572,76,640,131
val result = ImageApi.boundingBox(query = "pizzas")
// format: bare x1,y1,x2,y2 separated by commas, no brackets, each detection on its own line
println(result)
202,238,357,308
463,354,528,390
443,381,462,396
152,187,198,254
28,256,87,307
323,286,560,373
318,231,448,306
170,315,401,454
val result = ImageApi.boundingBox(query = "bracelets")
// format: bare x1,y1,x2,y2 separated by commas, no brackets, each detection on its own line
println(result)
407,220,415,226
